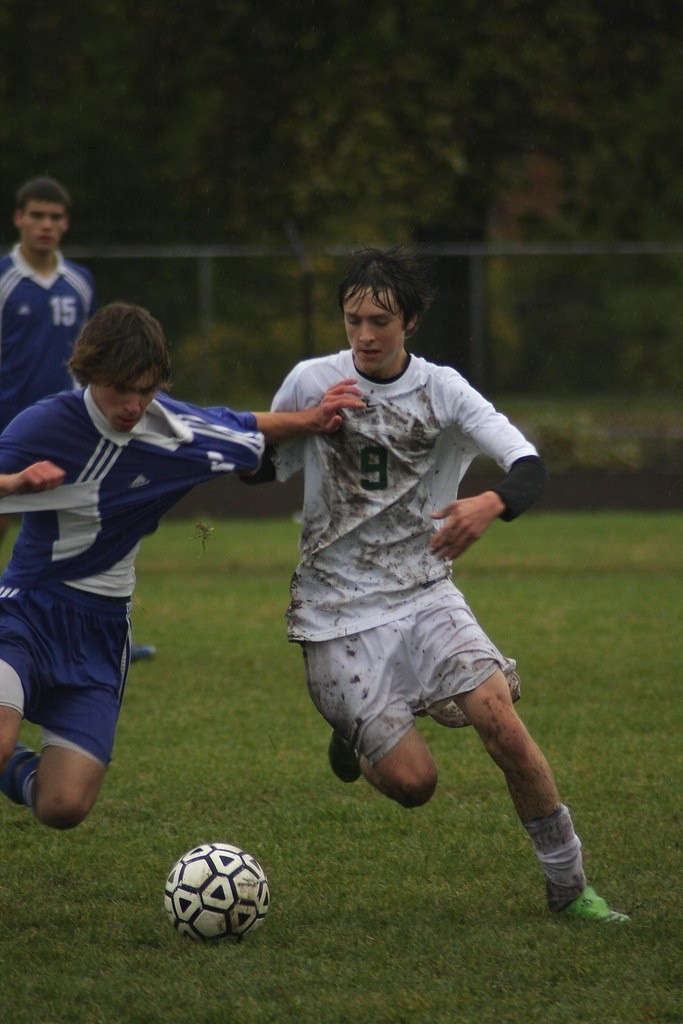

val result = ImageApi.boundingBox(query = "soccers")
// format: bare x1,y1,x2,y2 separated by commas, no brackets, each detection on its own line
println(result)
162,841,272,946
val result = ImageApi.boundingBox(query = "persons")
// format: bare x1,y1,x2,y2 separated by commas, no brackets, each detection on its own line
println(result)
0,304,366,830
0,177,156,664
234,246,632,924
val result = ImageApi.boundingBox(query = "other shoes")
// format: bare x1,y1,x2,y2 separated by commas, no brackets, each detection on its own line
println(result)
0,742,37,806
129,642,157,664
558,886,631,925
328,727,361,783
